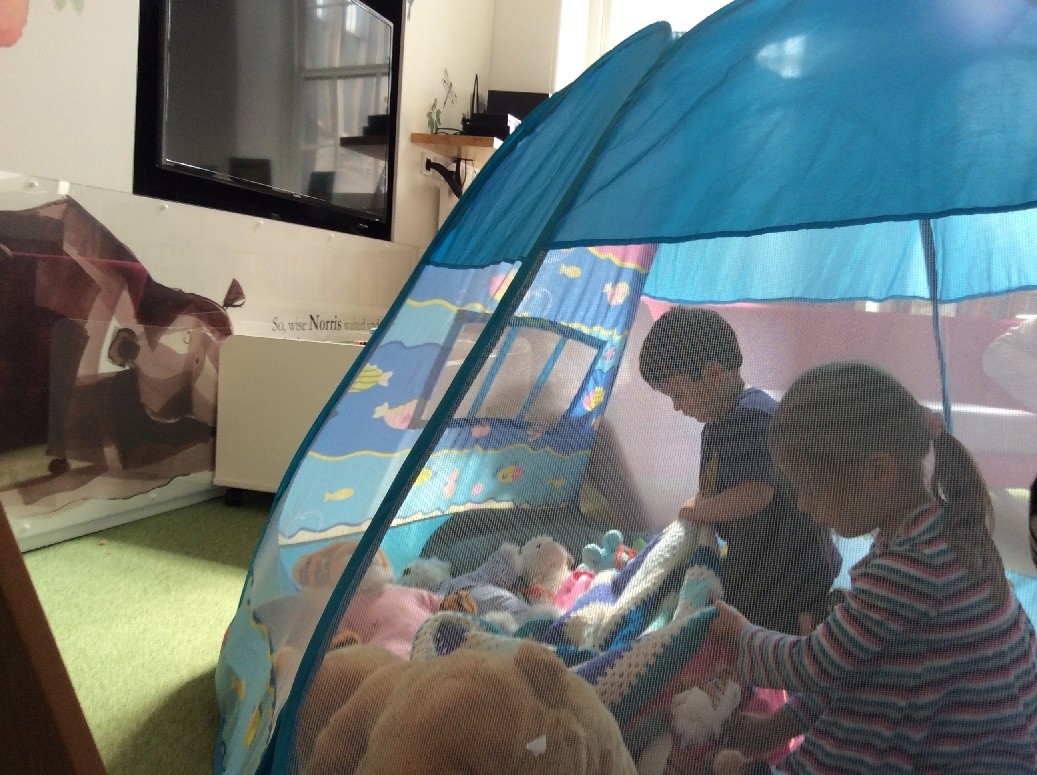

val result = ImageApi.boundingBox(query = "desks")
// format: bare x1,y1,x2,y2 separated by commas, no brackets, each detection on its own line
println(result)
214,335,531,506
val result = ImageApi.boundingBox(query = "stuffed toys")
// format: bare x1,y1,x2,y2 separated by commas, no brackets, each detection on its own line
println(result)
267,530,752,775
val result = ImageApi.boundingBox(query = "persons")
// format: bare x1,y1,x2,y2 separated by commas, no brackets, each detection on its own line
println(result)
641,307,854,635
698,361,1036,774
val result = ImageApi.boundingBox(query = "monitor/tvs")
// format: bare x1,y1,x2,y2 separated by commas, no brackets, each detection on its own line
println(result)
132,0,397,243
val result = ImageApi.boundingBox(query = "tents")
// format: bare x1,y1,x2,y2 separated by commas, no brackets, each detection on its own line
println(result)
212,2,1037,775
